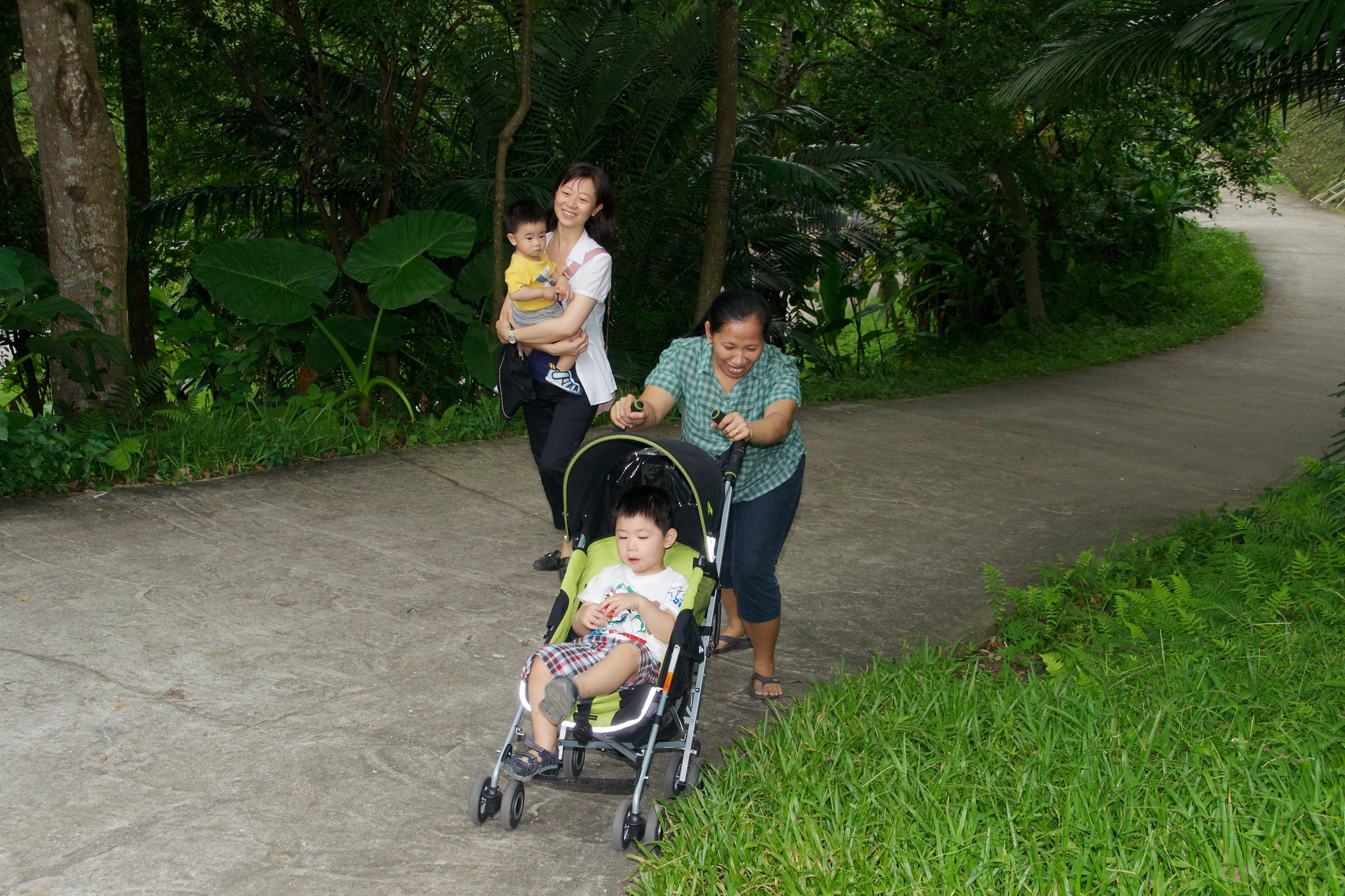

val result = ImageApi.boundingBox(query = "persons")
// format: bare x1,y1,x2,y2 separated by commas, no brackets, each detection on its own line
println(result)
610,289,808,700
502,198,582,394
495,162,617,580
501,487,689,783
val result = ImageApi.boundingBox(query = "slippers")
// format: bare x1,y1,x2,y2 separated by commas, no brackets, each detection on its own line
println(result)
711,635,752,654
749,671,780,700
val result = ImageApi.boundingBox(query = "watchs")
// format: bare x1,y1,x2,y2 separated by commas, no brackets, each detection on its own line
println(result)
508,328,517,344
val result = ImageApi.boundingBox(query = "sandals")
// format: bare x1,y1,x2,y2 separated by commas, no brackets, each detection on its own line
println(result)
499,737,558,783
545,363,583,396
493,385,498,394
538,675,579,725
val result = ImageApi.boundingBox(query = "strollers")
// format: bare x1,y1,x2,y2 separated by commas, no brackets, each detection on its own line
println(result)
468,399,747,857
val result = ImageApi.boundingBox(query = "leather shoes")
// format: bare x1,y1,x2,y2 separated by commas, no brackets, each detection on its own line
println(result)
533,550,562,571
559,556,571,582
